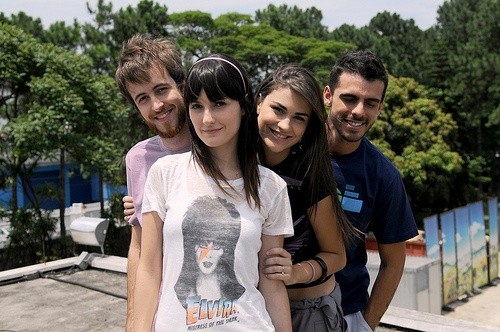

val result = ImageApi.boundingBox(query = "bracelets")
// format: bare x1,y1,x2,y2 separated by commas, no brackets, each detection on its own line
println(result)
309,256,327,284
301,261,315,284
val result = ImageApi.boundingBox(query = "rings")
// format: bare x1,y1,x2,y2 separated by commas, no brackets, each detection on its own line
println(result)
281,271,284,280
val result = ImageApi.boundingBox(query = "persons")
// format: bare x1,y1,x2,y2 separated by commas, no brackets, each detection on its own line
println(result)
122,63,348,332
114,34,192,332
323,51,419,332
132,53,293,332
